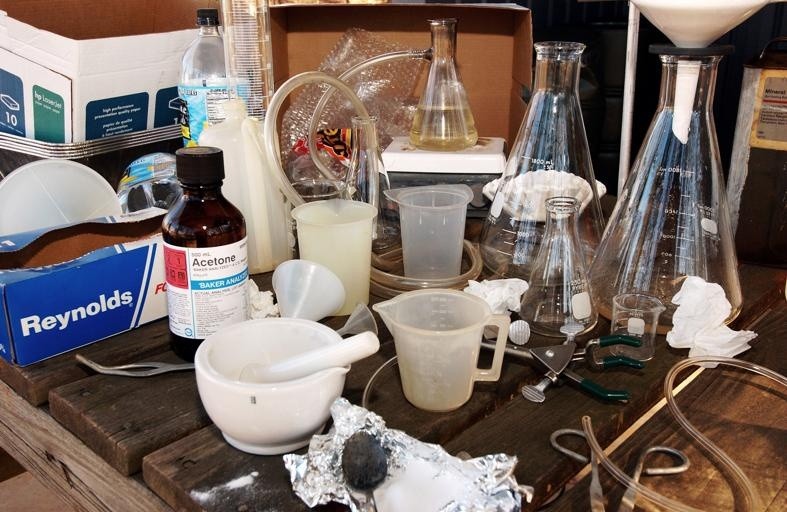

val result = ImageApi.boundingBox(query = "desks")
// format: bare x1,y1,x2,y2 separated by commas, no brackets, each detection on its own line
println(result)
0,243,786,512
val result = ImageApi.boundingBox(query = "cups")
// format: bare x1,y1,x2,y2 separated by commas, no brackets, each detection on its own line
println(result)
610,293,664,363
290,199,379,317
372,289,512,413
385,183,474,282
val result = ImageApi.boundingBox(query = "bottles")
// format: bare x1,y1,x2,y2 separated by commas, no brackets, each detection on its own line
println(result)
410,20,478,150
523,196,599,336
178,8,248,147
589,54,745,333
284,180,348,201
340,116,400,252
476,40,606,287
161,146,249,362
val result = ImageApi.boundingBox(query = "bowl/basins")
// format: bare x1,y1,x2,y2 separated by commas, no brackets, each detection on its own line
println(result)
194,318,351,455
